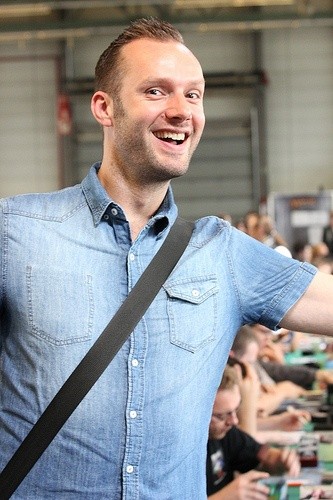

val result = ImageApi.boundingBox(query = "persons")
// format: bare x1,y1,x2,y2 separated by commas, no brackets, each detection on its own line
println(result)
205,209,333,500
0,17,333,500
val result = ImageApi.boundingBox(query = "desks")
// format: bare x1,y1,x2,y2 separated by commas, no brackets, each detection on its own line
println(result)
253,335,333,500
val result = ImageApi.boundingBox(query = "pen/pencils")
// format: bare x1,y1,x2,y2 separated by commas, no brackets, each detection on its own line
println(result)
288,483,314,487
286,405,312,431
